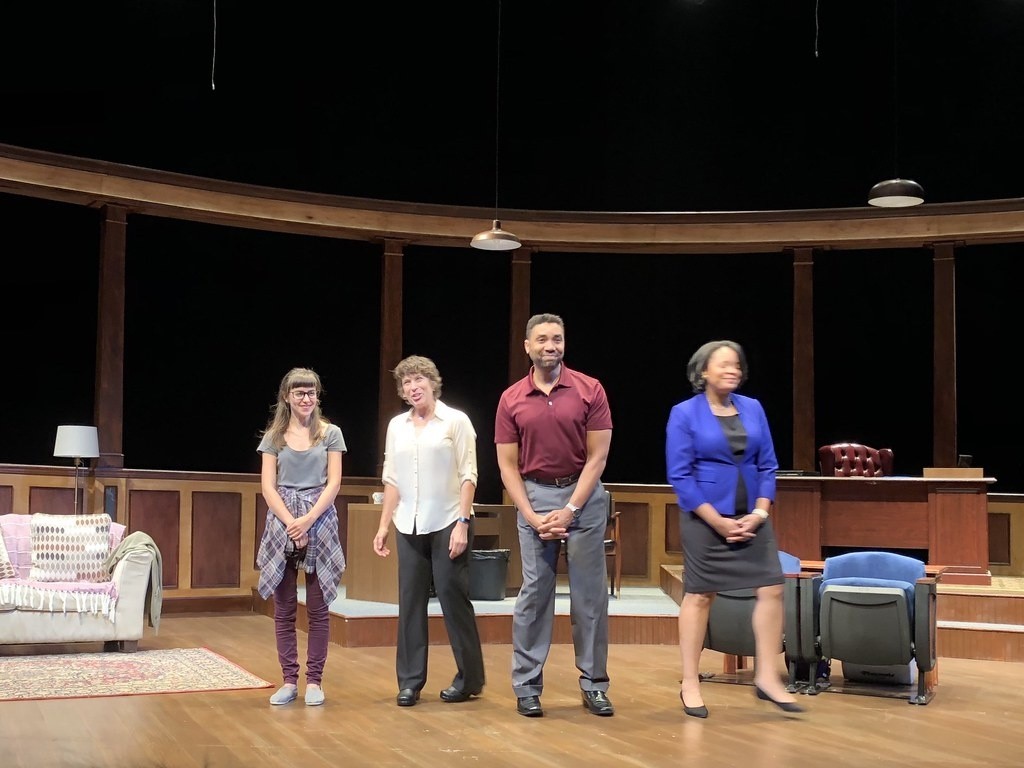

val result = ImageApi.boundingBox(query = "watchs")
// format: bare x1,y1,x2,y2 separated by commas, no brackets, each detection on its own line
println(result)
566,503,581,519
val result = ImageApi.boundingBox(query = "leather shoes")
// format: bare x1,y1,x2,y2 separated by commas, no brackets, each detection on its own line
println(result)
397,688,420,706
517,695,542,716
440,685,482,699
581,690,614,714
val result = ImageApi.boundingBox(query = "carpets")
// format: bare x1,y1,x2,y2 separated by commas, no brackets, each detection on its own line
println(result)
0,647,276,703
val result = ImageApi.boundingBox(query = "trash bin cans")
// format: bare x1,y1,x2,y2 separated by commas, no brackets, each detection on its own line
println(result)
465,547,510,601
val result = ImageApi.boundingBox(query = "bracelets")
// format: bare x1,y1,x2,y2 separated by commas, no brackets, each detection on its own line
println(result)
458,517,471,526
752,509,770,520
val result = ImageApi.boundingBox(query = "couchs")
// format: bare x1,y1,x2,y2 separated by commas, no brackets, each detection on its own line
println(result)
0,513,163,659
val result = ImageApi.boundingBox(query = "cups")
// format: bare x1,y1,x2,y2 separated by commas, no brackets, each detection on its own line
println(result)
373,492,384,505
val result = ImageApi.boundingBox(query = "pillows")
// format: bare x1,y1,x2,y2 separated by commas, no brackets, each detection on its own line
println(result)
29,511,112,584
0,530,15,580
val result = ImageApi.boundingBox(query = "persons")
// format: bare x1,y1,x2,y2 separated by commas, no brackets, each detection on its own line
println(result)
494,313,615,717
252,367,347,706
373,356,486,707
665,341,805,719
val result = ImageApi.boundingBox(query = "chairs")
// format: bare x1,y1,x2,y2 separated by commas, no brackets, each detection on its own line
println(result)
700,548,808,694
819,443,895,477
559,489,622,599
799,551,942,707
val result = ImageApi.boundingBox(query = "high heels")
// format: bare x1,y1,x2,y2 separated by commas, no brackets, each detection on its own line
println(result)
680,691,708,717
758,688,806,712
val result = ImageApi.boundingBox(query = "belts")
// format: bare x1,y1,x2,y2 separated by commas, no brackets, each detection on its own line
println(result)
527,472,580,488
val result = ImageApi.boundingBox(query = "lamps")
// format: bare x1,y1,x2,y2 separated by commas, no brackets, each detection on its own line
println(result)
53,424,101,514
867,0,926,208
469,0,522,252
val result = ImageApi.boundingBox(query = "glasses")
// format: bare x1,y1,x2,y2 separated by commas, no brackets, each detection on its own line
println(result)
289,390,319,399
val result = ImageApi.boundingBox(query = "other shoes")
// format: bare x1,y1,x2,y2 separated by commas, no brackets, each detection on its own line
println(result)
305,686,324,705
270,687,297,704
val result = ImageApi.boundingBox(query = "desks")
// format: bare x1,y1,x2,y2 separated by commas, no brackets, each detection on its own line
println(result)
800,561,949,694
770,476,997,585
346,503,523,605
